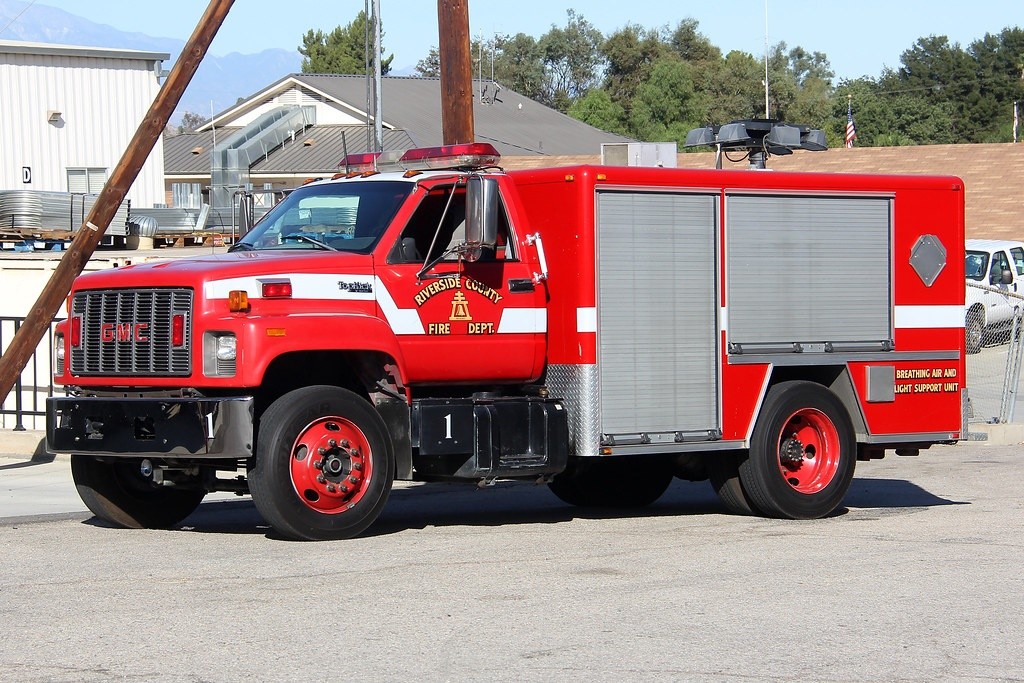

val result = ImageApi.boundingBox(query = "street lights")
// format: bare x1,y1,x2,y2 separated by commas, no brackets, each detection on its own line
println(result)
683,119,828,170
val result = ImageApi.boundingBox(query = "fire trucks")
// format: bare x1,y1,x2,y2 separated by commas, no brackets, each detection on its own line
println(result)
45,142,988,541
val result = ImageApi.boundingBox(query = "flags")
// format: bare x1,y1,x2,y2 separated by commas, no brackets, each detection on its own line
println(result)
847,106,856,148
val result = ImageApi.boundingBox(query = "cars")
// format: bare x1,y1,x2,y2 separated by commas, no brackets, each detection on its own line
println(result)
964,239,1024,354
